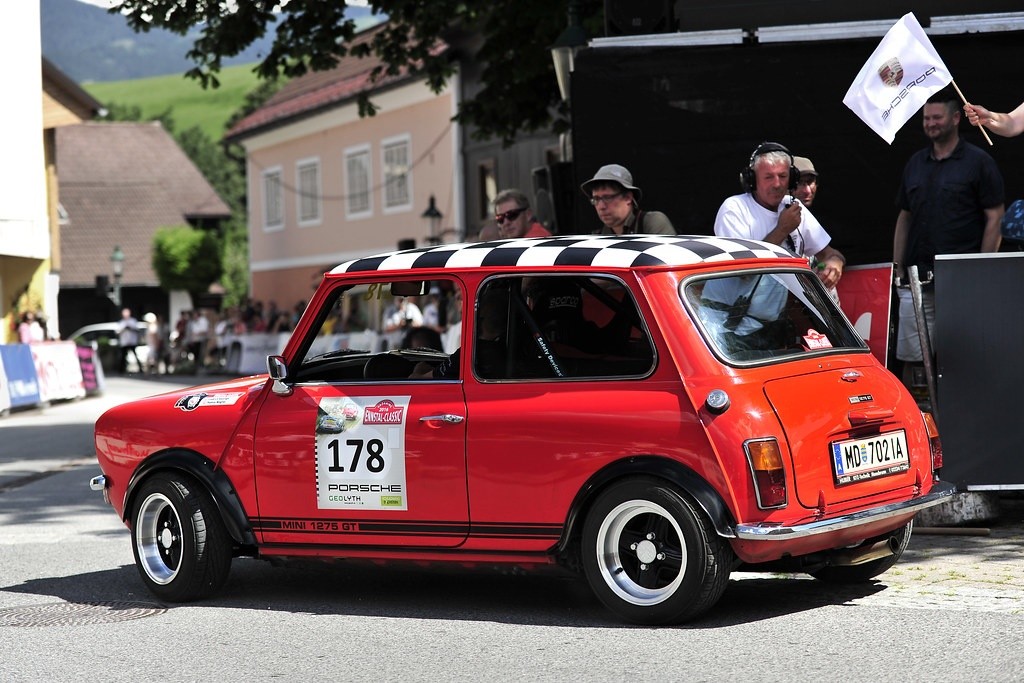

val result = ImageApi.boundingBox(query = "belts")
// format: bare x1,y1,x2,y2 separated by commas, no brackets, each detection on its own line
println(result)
700,297,770,327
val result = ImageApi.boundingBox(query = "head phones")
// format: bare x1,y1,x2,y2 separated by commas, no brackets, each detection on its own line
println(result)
739,142,801,193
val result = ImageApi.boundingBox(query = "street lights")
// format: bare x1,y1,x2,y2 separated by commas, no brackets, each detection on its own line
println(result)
109,245,125,318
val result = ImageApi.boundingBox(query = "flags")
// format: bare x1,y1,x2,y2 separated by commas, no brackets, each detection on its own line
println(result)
842,12,952,145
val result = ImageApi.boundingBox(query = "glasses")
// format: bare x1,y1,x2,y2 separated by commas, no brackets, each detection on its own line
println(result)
496,206,529,224
590,190,624,205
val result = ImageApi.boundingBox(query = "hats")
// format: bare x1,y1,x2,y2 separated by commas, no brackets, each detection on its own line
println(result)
428,287,440,295
790,155,818,176
581,164,642,209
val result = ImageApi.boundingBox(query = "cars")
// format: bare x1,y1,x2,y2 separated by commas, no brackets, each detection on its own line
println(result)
87,234,957,626
68,322,148,351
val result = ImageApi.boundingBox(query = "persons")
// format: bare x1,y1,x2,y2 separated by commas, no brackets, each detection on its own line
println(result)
492,187,553,296
114,308,209,374
382,288,458,350
479,220,502,241
444,290,462,355
963,102,1024,137
216,294,306,356
317,303,368,336
581,164,676,288
18,313,44,344
788,157,818,206
894,89,1005,370
698,142,845,354
409,348,460,379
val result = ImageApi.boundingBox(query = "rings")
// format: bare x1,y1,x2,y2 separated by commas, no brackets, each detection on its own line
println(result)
817,262,825,271
